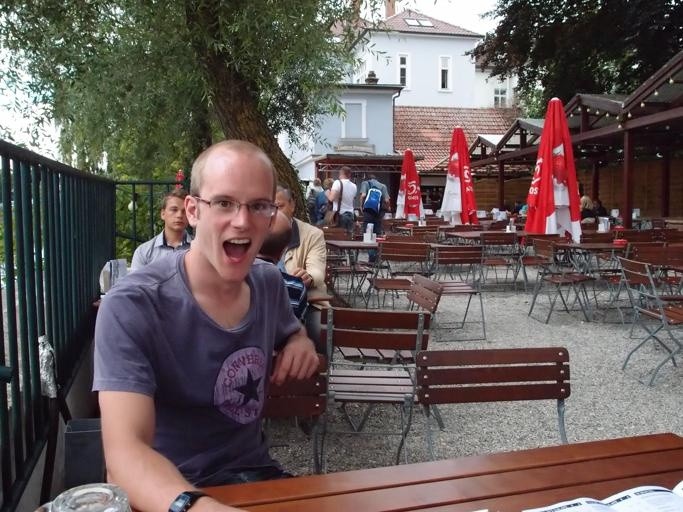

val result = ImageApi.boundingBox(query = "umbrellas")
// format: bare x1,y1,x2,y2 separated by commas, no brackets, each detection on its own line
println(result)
523,97,582,245
395,148,425,220
440,125,480,225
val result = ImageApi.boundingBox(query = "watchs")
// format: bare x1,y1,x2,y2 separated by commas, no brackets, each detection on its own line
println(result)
169,491,208,511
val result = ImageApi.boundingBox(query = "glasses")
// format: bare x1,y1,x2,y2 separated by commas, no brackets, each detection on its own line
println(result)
193,196,278,217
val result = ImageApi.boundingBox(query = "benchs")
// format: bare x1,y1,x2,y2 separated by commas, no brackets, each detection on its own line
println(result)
92,206,682,511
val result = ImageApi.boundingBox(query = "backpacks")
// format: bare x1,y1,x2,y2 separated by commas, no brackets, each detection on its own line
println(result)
362,181,384,217
305,189,317,215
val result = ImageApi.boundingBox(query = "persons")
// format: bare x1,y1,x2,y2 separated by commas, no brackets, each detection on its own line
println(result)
132,189,191,269
93,141,318,511
519,204,528,217
511,200,523,215
577,178,608,220
255,187,329,435
305,166,390,266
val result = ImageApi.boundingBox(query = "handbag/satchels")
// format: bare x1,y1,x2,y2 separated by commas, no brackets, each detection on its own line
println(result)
323,211,339,227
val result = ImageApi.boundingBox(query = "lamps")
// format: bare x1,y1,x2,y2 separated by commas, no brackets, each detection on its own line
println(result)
462,78,682,160
